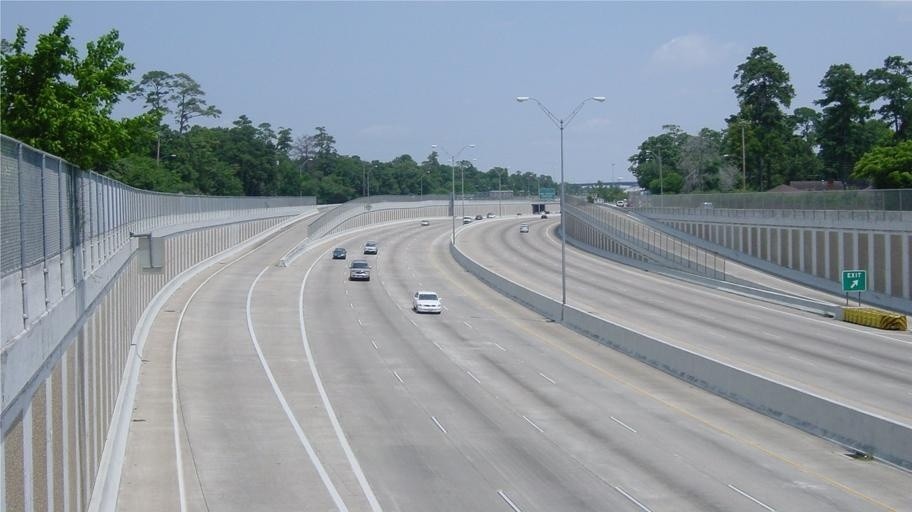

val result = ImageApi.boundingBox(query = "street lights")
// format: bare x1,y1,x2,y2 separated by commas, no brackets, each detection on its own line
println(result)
611,161,617,183
722,152,747,218
431,144,475,244
448,158,477,218
529,174,548,213
420,171,430,200
490,165,510,217
158,153,176,166
645,150,664,214
367,165,378,205
516,95,606,304
298,158,312,195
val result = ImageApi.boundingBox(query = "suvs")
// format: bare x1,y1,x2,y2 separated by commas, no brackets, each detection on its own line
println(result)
350,259,371,281
364,239,377,255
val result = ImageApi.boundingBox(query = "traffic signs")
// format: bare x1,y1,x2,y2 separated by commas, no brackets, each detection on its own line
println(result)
540,188,555,200
842,270,866,291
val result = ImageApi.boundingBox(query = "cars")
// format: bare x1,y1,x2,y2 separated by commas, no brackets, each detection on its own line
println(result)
420,220,429,227
616,201,624,207
463,210,549,233
333,248,347,259
413,289,443,315
699,202,712,213
